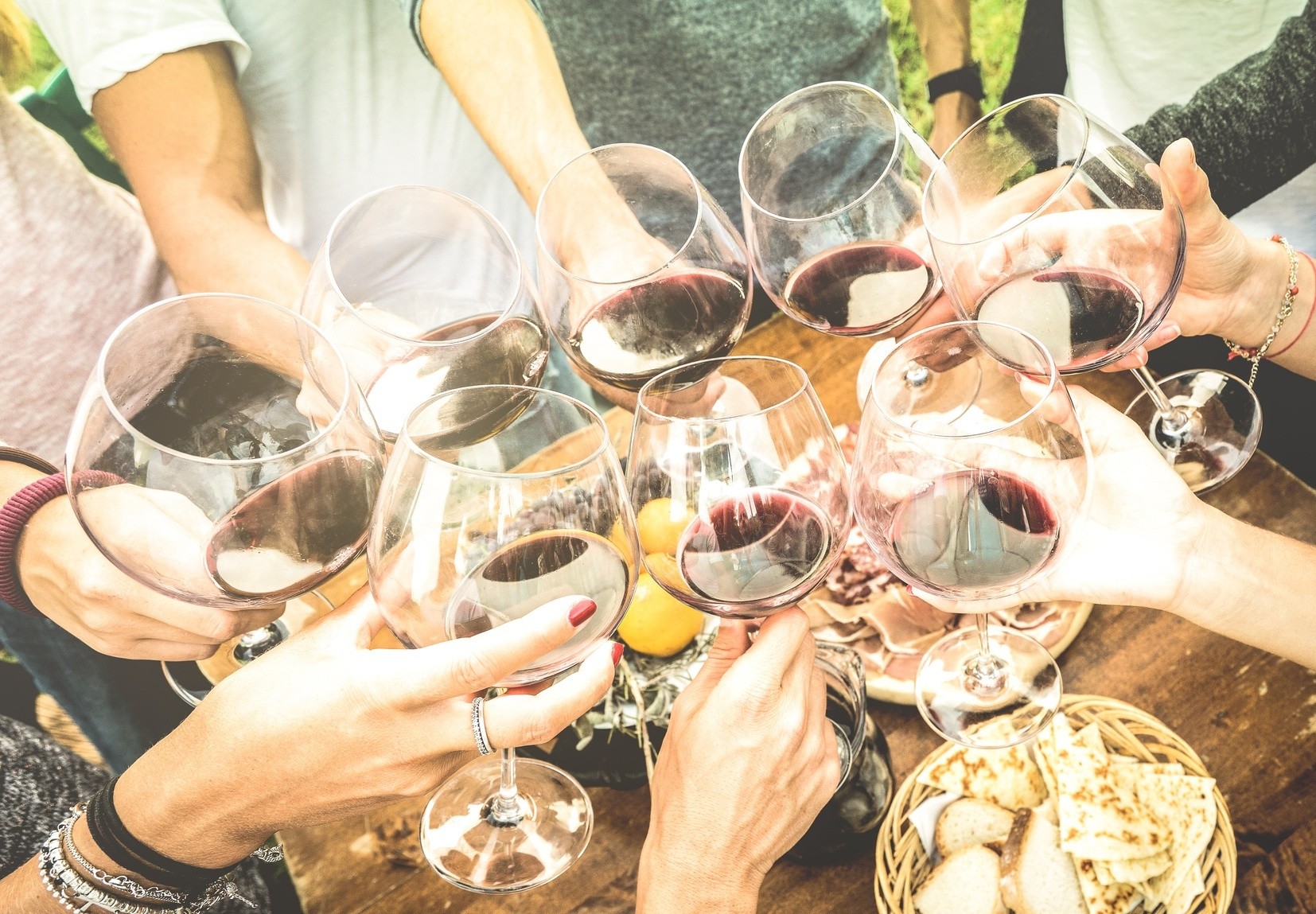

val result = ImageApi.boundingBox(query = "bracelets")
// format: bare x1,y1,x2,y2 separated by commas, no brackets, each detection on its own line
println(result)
1223,234,1299,389
1,468,124,617
1226,250,1316,358
926,63,985,103
38,777,284,914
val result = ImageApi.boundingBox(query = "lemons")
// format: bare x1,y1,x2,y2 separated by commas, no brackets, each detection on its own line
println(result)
604,494,710,657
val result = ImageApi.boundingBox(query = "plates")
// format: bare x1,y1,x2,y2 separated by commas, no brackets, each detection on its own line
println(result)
769,418,1092,708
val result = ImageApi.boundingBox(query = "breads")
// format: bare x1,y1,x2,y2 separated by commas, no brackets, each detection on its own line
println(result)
912,844,1011,914
1000,806,1090,914
934,797,1018,853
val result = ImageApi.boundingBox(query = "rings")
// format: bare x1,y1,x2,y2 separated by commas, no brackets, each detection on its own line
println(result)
474,695,494,755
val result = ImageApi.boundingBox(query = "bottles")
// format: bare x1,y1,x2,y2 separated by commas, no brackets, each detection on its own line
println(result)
925,95,1263,502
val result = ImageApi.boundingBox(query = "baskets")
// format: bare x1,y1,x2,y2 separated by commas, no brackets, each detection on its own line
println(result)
885,690,1238,914
773,434,1094,703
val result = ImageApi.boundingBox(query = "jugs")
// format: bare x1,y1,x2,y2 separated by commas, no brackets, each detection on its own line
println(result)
672,639,898,867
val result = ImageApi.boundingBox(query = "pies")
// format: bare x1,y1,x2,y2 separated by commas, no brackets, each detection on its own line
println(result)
914,710,1217,914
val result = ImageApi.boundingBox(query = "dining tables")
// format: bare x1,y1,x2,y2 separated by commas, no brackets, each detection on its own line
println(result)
196,277,1315,914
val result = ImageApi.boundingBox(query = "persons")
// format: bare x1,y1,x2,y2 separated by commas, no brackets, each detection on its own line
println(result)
880,0,1316,375
0,18,313,914
632,600,844,914
33,2,726,474
0,541,624,914
878,140,1316,669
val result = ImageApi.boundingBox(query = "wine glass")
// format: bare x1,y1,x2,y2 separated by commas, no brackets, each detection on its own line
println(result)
732,77,984,434
855,328,1086,748
362,382,643,896
624,348,867,814
286,186,550,547
63,291,403,711
536,139,760,481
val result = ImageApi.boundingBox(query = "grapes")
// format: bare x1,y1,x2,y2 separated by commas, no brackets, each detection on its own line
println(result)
461,468,625,575
630,459,676,510
